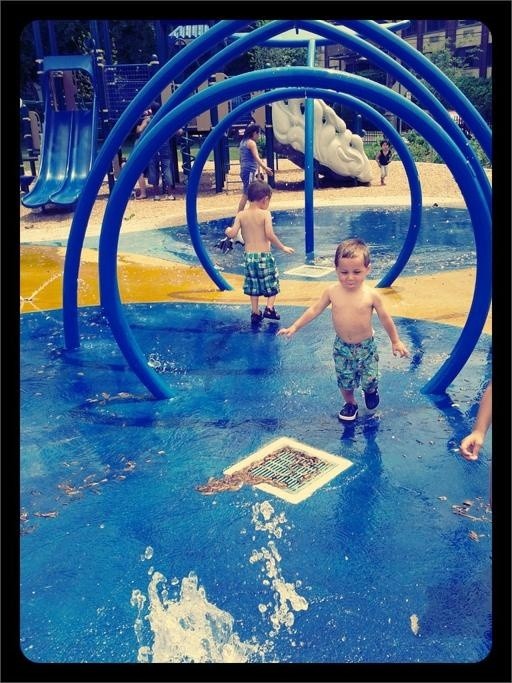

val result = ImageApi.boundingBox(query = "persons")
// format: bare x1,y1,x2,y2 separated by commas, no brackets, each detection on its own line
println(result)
224,180,296,322
276,239,408,419
134,102,184,199
459,380,492,463
376,141,392,185
238,124,273,210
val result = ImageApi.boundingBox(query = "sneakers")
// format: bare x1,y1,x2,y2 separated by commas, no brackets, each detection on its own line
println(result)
264,305,280,320
251,310,263,323
339,402,358,420
365,387,379,410
